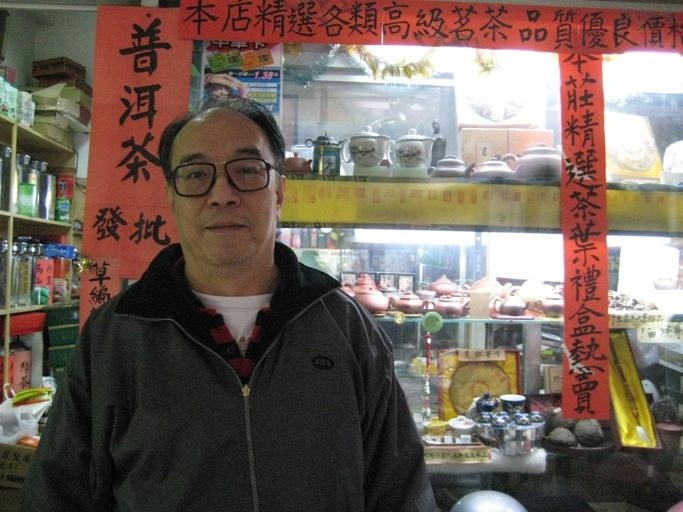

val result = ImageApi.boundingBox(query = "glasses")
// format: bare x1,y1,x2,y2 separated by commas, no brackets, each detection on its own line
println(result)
166,158,284,198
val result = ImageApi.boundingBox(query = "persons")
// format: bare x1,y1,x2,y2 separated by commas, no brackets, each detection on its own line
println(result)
26,93,448,510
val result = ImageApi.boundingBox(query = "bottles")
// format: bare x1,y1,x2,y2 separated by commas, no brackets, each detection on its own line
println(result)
0,144,77,310
278,227,336,250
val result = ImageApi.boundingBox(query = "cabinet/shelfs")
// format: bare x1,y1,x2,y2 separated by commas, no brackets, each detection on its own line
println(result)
0,113,79,407
172,40,682,445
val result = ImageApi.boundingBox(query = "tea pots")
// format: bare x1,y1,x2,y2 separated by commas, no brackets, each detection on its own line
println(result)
337,270,564,320
284,125,561,185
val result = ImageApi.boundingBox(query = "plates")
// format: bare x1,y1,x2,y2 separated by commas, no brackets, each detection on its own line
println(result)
448,361,512,417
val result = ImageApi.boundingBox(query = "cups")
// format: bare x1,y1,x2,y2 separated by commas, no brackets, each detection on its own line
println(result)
421,416,449,437
651,419,683,459
447,414,475,438
658,170,683,189
474,391,545,456
